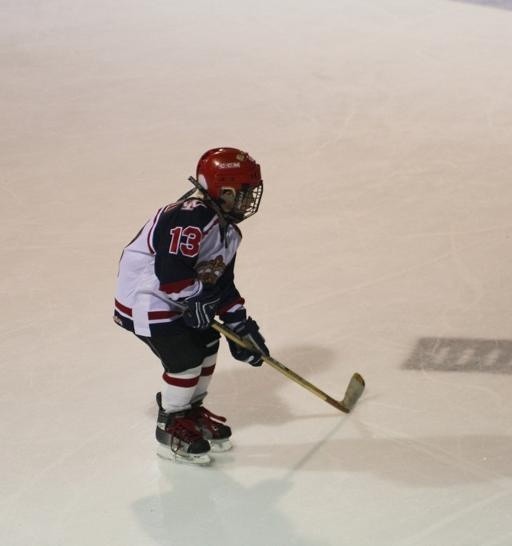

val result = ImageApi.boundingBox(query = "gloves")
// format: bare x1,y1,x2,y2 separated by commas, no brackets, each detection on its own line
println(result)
220,309,269,367
182,283,223,332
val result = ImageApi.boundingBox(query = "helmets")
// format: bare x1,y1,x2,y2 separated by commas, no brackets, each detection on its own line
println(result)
195,147,263,224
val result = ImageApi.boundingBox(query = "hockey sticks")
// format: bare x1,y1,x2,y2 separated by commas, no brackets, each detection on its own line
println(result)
211,320,364,412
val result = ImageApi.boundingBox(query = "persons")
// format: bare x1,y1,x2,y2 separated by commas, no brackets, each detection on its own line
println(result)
113,146,269,458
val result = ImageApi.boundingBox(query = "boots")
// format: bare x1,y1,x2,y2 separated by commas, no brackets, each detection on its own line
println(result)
154,392,210,454
190,390,232,441
